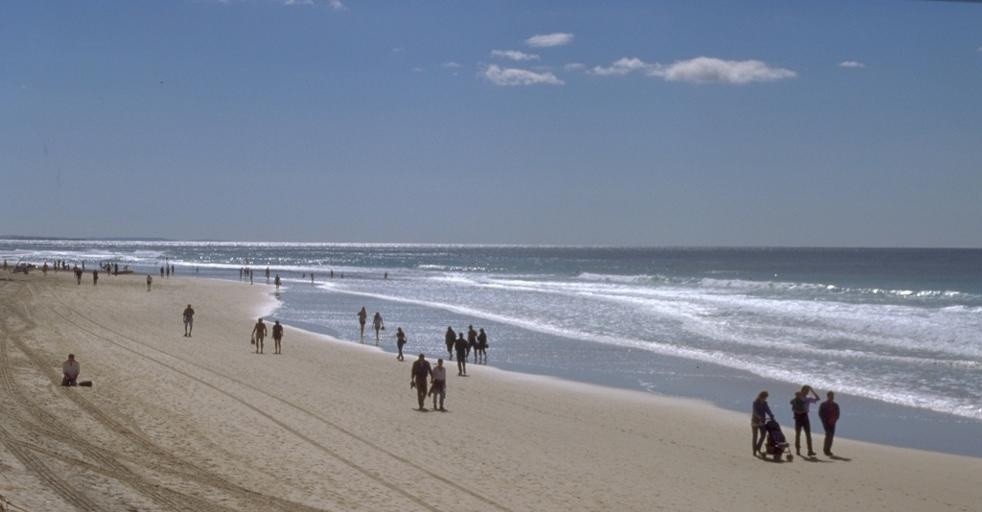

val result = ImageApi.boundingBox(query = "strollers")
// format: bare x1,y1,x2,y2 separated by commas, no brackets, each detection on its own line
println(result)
758,417,793,462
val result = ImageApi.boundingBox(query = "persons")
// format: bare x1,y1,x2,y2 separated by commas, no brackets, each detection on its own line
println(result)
230,260,283,291
818,391,840,455
14,260,175,290
182,303,195,337
751,390,774,456
790,385,820,457
349,296,487,410
272,320,283,354
60,354,80,386
250,318,267,354
